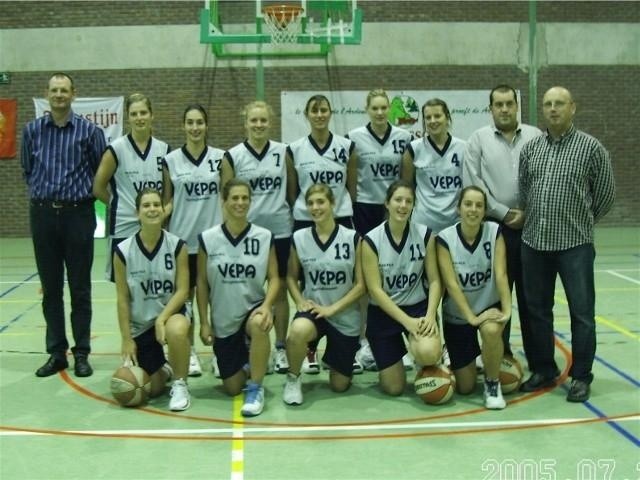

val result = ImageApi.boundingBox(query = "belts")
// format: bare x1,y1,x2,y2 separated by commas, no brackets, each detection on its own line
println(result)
32,199,81,209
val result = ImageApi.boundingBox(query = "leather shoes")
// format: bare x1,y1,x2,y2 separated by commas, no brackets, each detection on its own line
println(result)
566,381,590,403
36,356,69,377
74,355,93,376
519,372,560,392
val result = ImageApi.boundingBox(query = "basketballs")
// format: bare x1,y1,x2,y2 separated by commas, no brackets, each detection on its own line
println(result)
497,353,524,393
414,364,458,406
109,366,153,408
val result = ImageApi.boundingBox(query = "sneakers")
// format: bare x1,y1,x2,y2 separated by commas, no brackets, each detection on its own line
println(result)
169,380,192,412
482,379,506,409
403,354,412,368
475,351,485,372
273,349,289,372
211,358,221,378
284,373,303,406
302,350,320,374
188,351,203,375
351,358,362,375
241,384,266,417
442,347,451,368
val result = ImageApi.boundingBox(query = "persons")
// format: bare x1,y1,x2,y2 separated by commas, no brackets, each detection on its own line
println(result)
461,84,543,359
281,184,367,406
435,185,513,411
161,103,226,377
516,85,617,406
402,98,467,367
362,179,442,397
220,101,297,377
286,95,364,375
94,93,171,364
343,88,412,370
196,179,281,417
19,73,107,378
112,188,195,412
